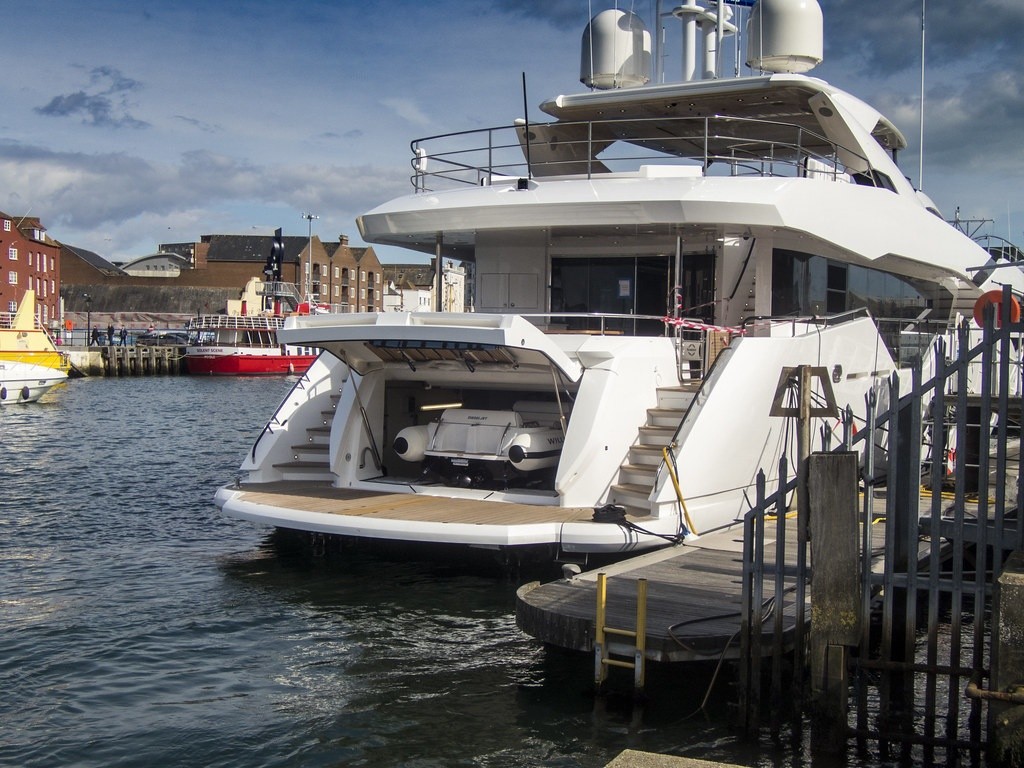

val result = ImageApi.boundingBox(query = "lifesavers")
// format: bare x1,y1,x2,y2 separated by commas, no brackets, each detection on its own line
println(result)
972,289,1021,328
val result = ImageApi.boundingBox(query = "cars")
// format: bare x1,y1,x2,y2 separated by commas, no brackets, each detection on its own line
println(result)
136,329,187,346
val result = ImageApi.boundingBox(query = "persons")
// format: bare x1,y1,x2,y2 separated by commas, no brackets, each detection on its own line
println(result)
119,325,128,347
89,325,99,346
107,322,114,346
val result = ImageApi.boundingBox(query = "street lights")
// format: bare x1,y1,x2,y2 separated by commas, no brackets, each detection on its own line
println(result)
84,295,94,347
302,212,319,301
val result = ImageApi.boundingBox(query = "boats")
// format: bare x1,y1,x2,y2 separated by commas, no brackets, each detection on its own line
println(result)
184,277,331,375
211,1,1024,567
391,396,574,470
0,289,73,404
